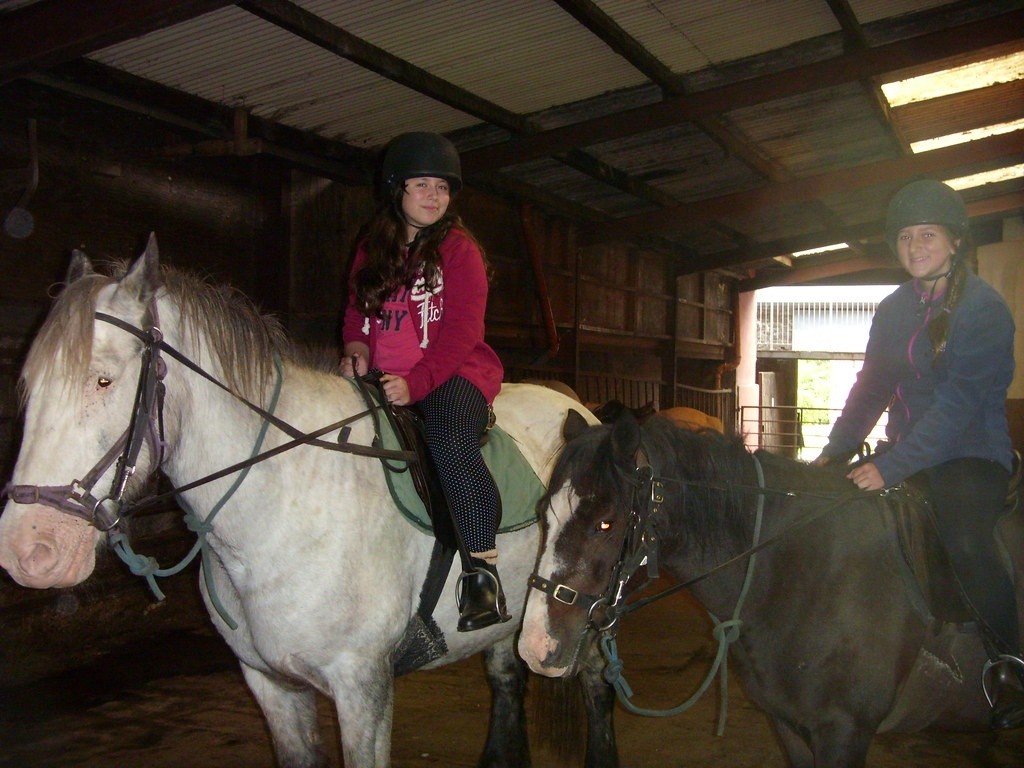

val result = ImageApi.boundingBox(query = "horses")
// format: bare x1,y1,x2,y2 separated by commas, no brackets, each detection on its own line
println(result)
517,407,1023,768
1,231,620,768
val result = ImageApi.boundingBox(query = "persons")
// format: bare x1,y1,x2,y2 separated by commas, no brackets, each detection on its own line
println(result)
809,181,1024,730
338,132,508,632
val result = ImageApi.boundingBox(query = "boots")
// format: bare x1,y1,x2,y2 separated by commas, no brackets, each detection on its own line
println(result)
989,661,1024,732
457,565,507,633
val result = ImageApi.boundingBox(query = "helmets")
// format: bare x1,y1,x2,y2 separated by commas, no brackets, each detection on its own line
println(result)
884,179,965,258
374,132,462,203
